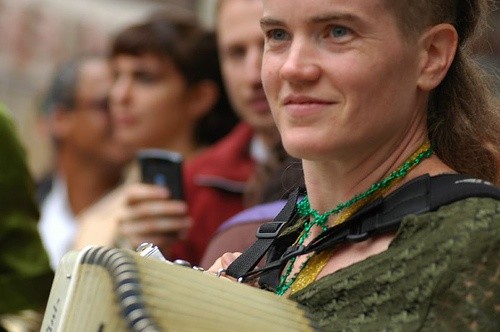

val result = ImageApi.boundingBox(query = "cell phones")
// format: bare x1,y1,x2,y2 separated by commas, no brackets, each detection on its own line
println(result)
136,148,184,201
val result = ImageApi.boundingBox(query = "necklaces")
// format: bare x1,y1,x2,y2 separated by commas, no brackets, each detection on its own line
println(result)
273,141,435,296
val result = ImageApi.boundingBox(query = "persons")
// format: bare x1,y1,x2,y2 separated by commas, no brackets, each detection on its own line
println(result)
112,14,234,184
32,56,124,272
69,1,292,269
258,1,499,332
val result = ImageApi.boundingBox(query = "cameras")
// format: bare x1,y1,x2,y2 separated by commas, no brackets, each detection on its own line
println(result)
135,242,204,271
